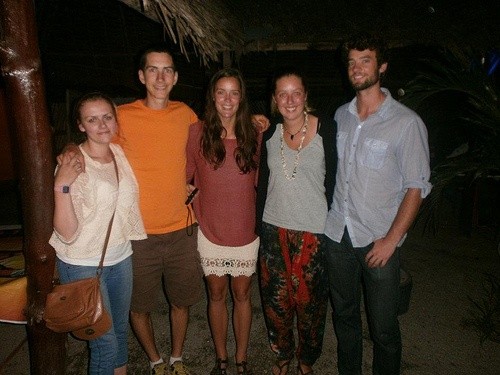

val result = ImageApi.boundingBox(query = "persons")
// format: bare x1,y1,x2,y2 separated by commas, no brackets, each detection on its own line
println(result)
322,36,434,375
47,91,199,375
184,67,263,375
255,69,339,375
56,46,271,375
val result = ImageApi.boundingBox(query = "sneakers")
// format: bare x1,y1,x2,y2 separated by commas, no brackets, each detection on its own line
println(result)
168,358,187,375
150,362,167,375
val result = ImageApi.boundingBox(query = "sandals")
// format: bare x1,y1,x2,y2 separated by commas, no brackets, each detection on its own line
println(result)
235,353,249,375
210,358,228,375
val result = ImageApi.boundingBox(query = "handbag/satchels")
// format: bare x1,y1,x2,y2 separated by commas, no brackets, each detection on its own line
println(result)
42,276,112,341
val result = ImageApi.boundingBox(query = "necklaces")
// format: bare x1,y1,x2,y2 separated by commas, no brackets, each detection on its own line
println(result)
280,111,308,183
284,115,306,140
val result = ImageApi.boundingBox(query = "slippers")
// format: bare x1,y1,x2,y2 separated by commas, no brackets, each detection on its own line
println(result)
272,359,289,375
297,361,313,375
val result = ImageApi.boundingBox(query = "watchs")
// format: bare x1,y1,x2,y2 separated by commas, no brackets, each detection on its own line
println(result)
53,185,70,193
